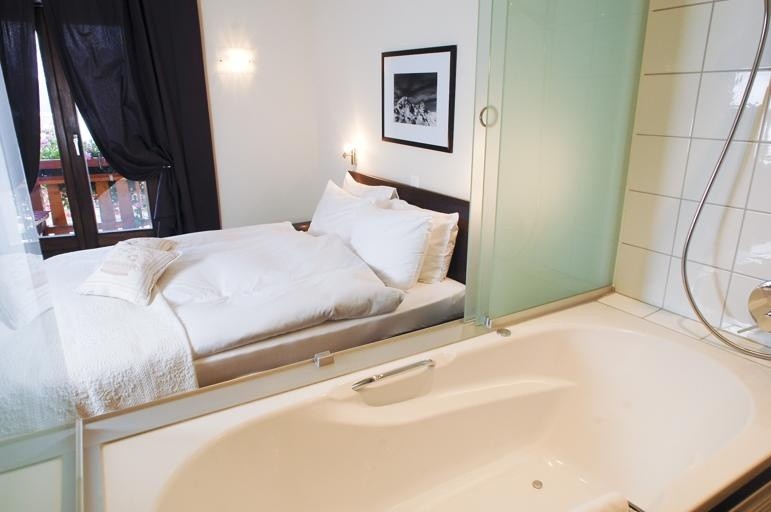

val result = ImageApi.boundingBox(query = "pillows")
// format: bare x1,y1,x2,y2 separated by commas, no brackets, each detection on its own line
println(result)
76,236,183,311
306,170,460,291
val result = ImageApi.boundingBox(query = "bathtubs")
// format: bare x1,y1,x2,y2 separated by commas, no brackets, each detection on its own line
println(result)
100,301,771,512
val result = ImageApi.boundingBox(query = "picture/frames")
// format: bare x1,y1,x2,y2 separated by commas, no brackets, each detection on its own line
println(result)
380,43,458,154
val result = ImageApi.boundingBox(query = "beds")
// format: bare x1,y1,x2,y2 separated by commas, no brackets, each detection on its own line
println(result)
42,169,472,421
